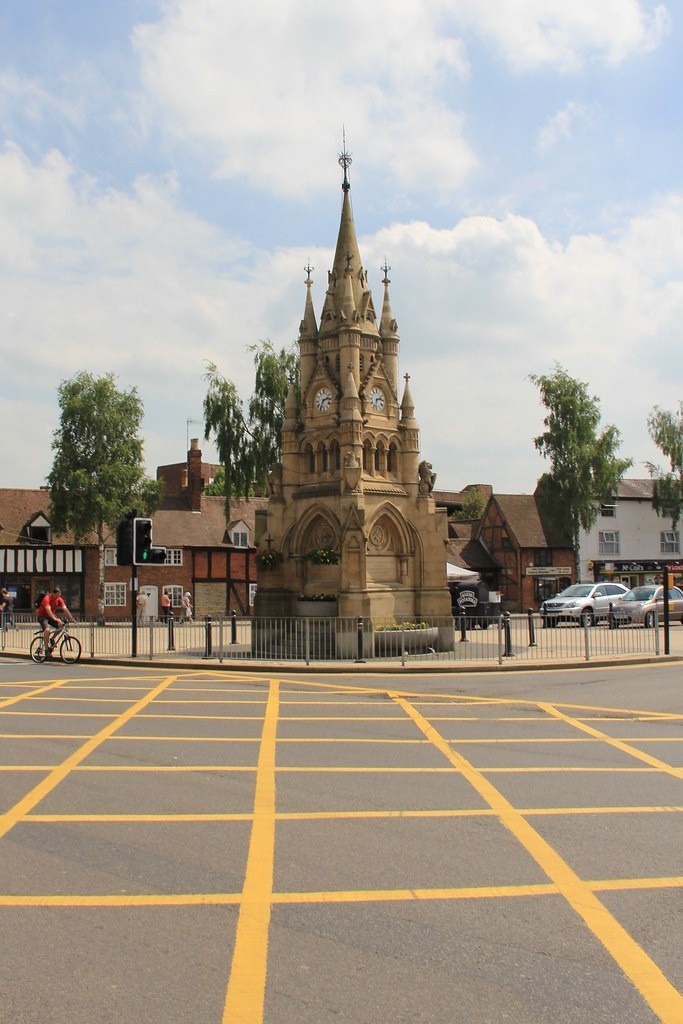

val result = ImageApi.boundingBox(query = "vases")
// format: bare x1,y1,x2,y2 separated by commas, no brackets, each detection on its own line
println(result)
296,601,338,617
374,627,439,650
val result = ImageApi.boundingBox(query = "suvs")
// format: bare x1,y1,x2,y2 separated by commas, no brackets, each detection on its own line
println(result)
538,582,636,627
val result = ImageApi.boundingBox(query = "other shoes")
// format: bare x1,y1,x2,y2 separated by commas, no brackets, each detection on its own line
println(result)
50,638,57,647
45,649,51,659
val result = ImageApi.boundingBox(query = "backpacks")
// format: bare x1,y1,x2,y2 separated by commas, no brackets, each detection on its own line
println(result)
34,591,60,610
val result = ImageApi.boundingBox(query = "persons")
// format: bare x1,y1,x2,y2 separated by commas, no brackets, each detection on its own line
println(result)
37,588,77,656
136,591,148,627
179,592,195,624
0,588,20,632
162,590,170,623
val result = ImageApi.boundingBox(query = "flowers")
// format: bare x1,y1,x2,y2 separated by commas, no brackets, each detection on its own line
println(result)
252,547,284,573
304,544,339,565
297,592,336,601
375,621,431,631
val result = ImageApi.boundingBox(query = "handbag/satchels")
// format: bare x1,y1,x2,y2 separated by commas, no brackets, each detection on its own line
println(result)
186,608,192,616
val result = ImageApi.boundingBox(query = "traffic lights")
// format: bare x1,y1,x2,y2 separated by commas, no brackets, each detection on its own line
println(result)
151,548,166,564
133,519,153,565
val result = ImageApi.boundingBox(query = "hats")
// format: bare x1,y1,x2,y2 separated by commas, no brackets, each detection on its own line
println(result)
185,592,192,597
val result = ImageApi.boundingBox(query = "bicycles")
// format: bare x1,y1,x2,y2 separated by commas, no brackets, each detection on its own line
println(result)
29,620,82,665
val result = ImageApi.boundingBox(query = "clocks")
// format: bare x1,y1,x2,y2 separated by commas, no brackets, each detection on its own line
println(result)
368,387,385,413
314,388,333,414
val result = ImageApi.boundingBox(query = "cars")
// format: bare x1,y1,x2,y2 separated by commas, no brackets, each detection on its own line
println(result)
607,584,683,628
